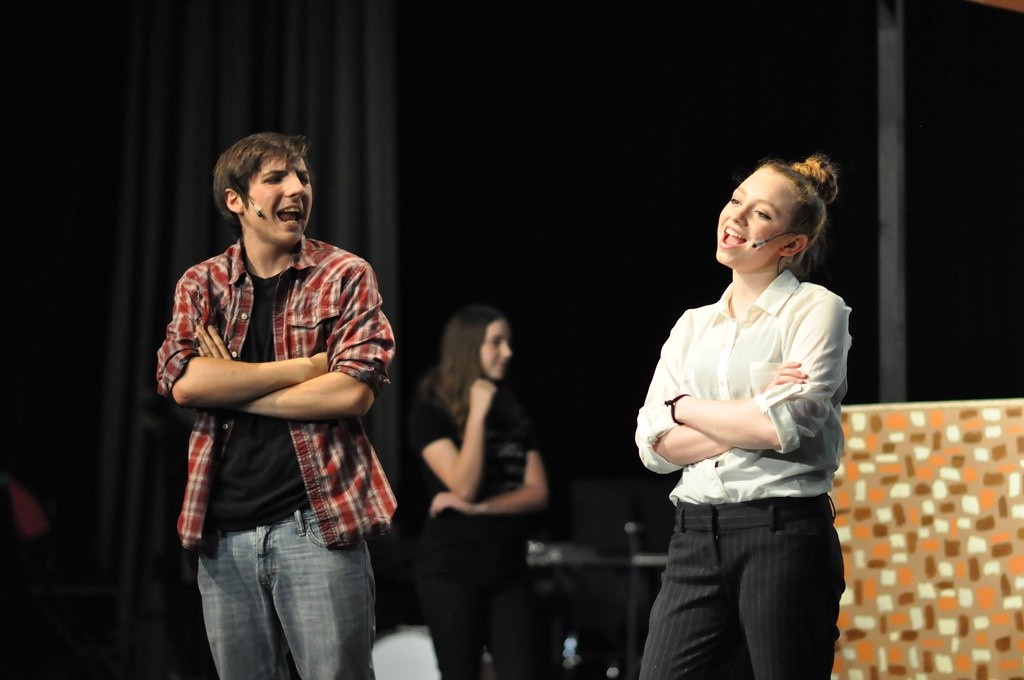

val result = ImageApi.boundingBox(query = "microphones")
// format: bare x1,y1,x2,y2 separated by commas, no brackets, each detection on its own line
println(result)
246,197,263,217
752,231,796,249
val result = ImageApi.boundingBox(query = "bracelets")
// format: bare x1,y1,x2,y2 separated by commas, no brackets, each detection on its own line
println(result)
664,394,690,425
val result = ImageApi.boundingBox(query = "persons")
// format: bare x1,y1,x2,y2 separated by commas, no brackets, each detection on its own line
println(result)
408,304,550,680
635,155,852,680
156,133,398,680
